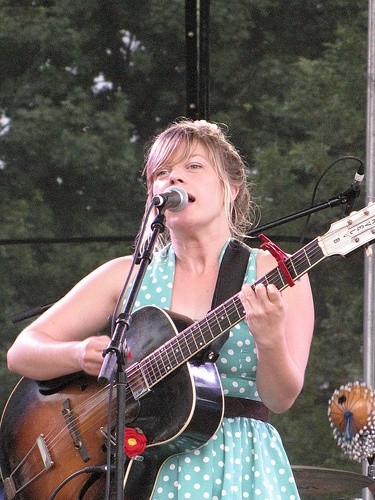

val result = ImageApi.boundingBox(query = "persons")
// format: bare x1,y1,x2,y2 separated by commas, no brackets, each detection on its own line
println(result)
7,119,314,500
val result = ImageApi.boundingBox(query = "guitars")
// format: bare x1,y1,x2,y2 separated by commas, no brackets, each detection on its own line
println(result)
0,202,375,500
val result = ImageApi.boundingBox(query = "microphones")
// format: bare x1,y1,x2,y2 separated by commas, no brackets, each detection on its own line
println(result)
150,184,190,212
342,163,365,219
